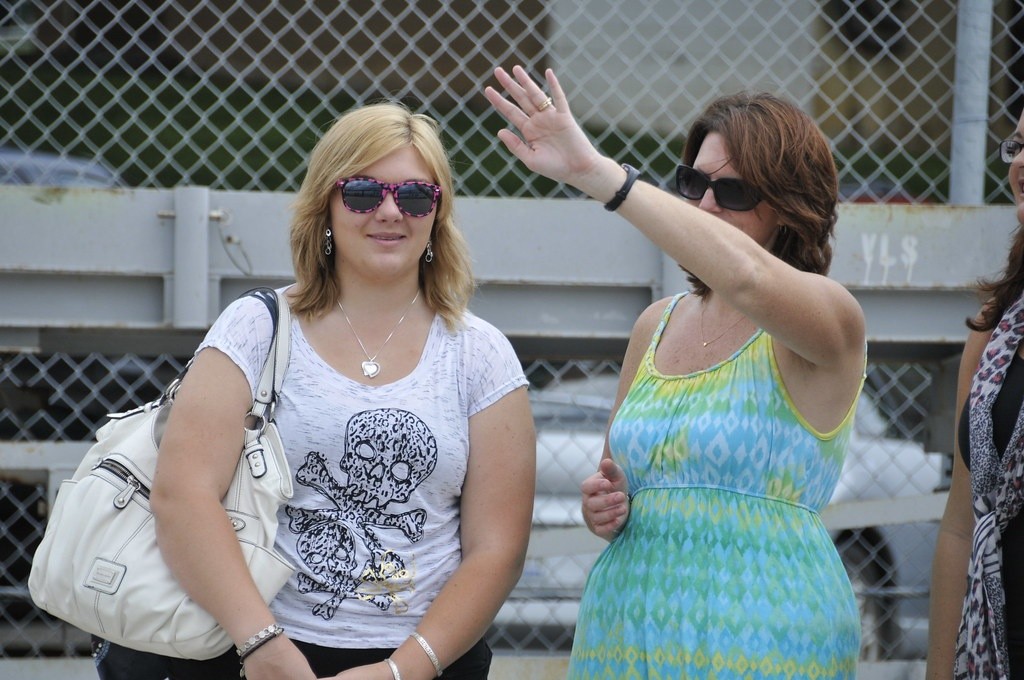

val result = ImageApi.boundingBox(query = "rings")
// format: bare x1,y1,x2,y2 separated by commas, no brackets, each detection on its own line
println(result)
536,97,552,112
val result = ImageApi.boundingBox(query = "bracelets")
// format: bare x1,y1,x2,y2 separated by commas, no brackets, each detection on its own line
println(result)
237,623,284,678
384,658,402,680
408,631,444,678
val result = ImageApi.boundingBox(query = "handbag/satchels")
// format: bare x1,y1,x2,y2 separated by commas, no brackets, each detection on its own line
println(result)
34,287,299,661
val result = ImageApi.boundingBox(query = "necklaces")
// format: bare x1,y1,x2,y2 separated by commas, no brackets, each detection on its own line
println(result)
700,294,745,347
335,287,421,378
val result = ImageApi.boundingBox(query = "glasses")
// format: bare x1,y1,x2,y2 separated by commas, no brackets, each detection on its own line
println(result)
999,140,1024,163
337,175,443,218
675,163,768,213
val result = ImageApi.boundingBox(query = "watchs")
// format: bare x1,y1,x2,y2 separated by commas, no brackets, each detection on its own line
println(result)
603,163,641,212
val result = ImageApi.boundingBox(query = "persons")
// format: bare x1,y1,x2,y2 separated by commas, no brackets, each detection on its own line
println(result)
921,106,1024,680
150,103,536,680
483,65,869,680
